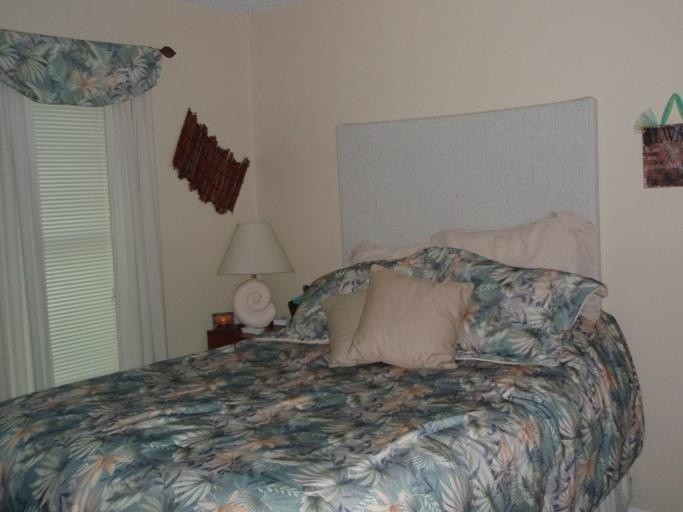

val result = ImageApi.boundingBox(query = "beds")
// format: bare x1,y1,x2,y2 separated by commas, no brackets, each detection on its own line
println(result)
0,230,648,511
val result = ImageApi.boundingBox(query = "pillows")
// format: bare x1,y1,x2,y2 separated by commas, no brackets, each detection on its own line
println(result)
318,286,368,368
252,255,410,345
346,263,475,370
394,246,608,368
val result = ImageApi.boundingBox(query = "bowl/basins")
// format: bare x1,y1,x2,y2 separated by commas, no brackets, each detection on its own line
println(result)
213,313,233,326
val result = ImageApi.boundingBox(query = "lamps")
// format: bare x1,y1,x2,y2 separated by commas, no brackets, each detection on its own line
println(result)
214,223,296,335
